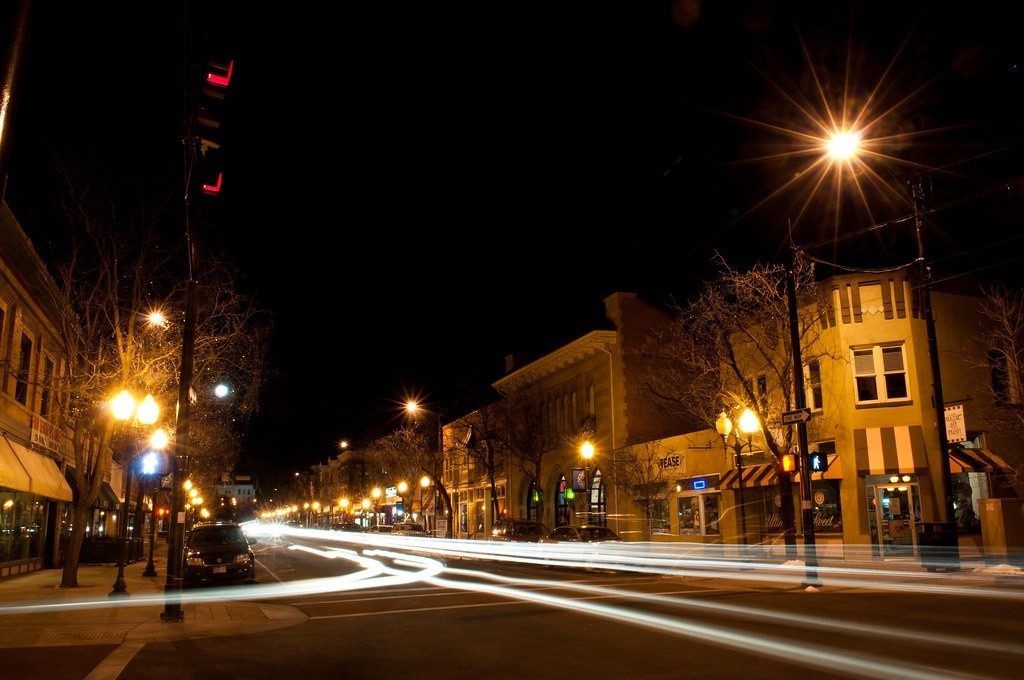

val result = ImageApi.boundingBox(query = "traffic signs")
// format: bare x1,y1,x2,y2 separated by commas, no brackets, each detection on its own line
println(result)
781,407,813,426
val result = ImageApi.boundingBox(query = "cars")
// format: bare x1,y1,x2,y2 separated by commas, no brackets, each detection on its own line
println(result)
538,524,625,543
331,523,362,532
491,518,550,543
366,524,393,534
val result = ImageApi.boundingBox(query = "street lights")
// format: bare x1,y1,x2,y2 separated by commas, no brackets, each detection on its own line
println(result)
109,389,160,596
579,440,594,496
780,127,864,587
140,429,168,577
716,408,761,545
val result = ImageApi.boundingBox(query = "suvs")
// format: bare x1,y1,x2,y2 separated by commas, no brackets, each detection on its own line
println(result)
391,522,425,537
183,520,256,587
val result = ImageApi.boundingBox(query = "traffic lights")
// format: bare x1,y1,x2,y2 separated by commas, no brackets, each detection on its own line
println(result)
191,53,233,200
783,454,799,473
809,451,828,471
